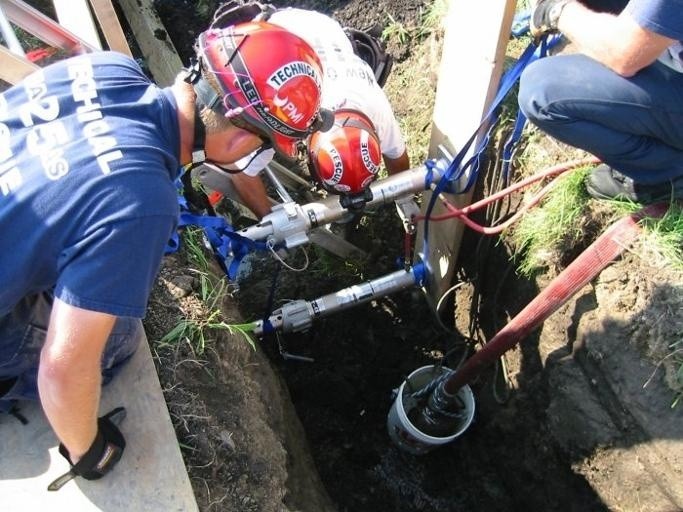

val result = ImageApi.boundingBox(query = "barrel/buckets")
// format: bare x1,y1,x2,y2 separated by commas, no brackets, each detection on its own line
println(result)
387,365,474,455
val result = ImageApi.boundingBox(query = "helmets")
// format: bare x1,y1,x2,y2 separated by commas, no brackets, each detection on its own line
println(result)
307,109,381,196
198,22,324,160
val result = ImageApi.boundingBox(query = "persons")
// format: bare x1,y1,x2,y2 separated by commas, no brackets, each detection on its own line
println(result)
516,0,682,208
205,3,422,262
0,19,324,479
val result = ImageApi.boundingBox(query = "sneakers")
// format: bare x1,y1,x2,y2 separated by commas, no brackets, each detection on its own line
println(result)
587,163,683,204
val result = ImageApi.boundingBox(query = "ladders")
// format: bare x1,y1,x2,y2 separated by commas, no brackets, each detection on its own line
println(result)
0,0,390,276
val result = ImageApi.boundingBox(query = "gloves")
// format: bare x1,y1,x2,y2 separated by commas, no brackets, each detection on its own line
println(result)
529,0,569,46
57,418,125,481
395,194,420,236
